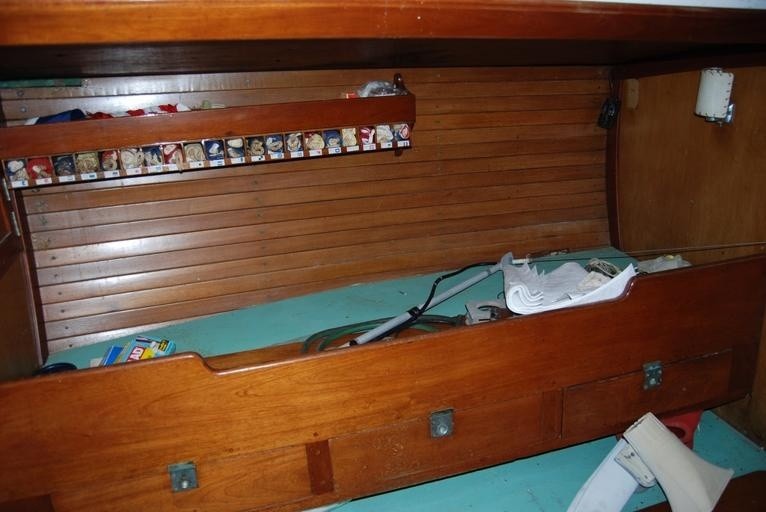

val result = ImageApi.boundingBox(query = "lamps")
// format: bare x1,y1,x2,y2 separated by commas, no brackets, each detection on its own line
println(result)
692,66,737,125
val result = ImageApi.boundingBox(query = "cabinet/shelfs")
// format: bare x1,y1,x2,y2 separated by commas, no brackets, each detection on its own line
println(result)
0,243,766,511
